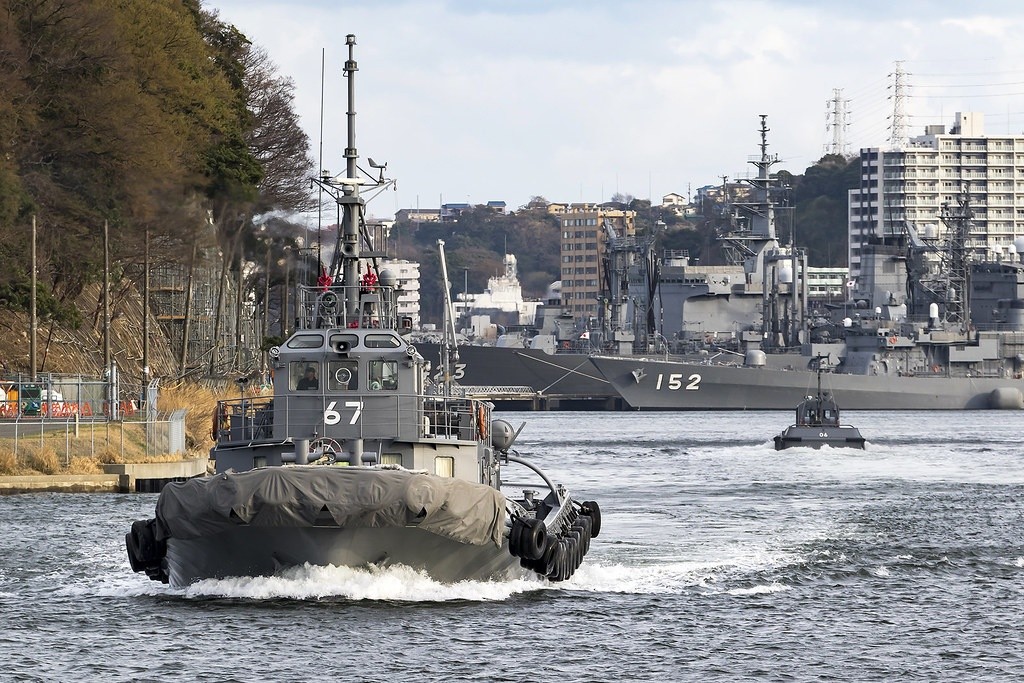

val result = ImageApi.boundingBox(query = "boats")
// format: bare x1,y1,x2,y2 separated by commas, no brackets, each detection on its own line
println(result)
773,350,867,455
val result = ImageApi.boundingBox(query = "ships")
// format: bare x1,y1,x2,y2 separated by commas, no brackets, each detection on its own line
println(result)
394,114,1022,410
124,34,604,595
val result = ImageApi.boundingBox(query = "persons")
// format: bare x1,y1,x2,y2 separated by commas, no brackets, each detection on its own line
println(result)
296,367,319,390
